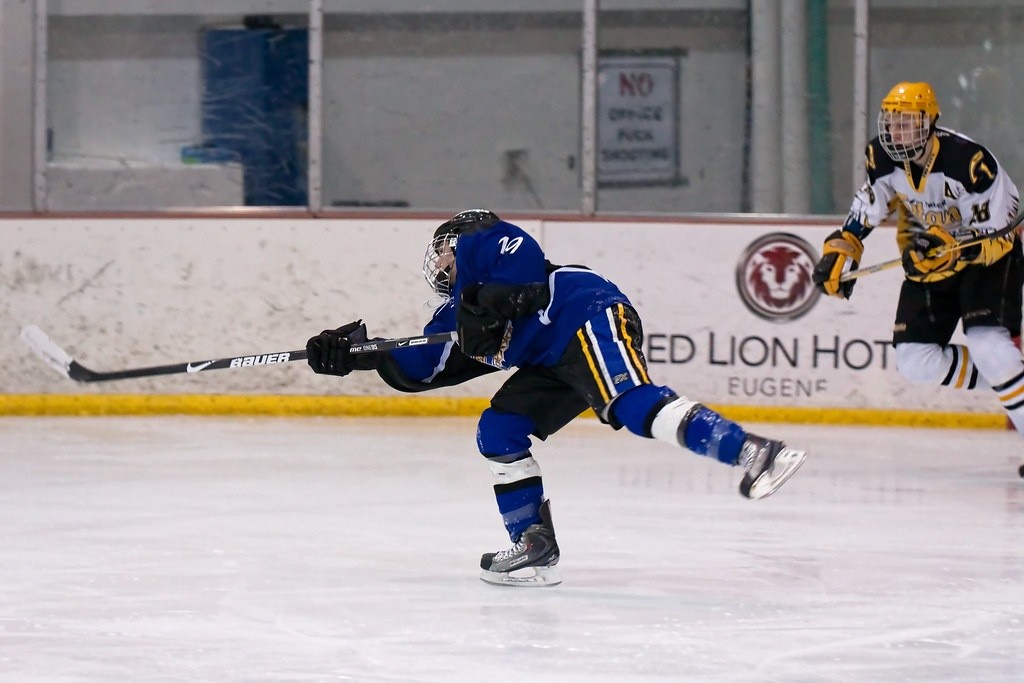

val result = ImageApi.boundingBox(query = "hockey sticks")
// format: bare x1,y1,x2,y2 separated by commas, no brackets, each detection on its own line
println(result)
20,323,458,388
836,209,1024,282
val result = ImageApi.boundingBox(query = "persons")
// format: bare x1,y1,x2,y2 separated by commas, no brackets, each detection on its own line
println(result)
307,209,806,586
811,81,1024,480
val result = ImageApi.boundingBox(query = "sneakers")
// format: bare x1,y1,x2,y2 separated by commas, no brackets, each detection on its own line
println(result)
737,433,805,501
480,498,560,585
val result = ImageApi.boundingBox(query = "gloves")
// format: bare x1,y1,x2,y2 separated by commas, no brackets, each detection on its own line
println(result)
812,231,864,300
455,282,517,357
305,319,368,377
902,226,970,284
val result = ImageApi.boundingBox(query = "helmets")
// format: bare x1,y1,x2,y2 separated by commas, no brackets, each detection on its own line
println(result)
879,82,940,160
422,208,499,296
